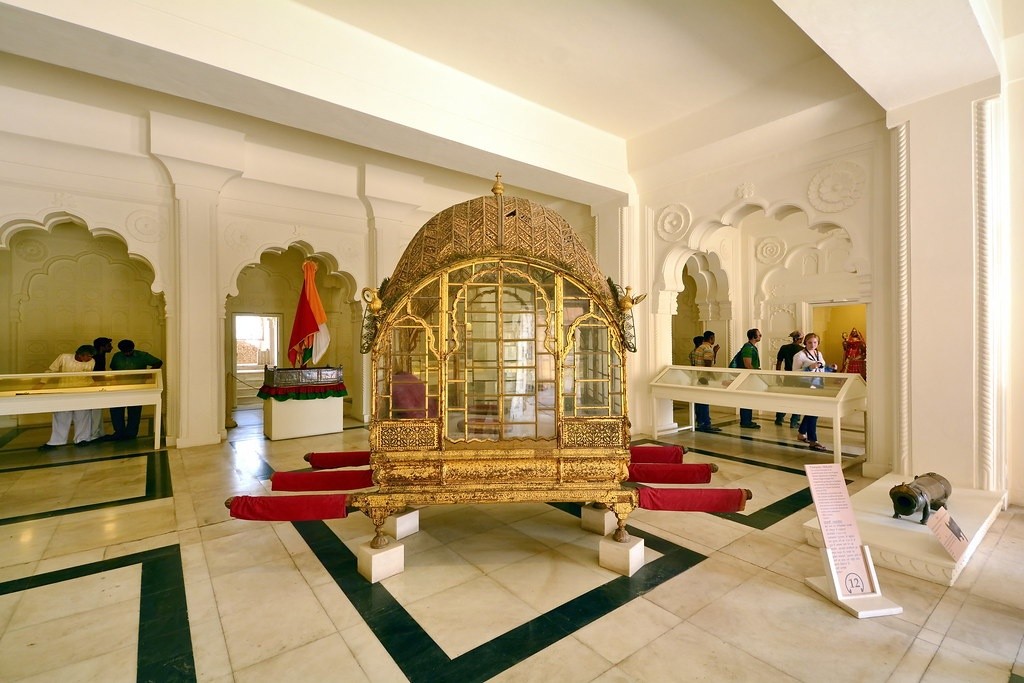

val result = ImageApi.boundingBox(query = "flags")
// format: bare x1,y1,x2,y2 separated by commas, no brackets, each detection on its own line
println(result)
287,262,331,368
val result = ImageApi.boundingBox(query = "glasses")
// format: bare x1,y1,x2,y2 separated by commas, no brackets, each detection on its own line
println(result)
758,335,761,337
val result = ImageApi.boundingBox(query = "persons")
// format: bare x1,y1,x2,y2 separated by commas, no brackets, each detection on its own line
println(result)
110,340,163,440
728,329,761,429
840,327,866,381
689,331,722,434
774,330,804,428
91,337,116,443
473,345,502,434
792,333,837,450
32,345,100,451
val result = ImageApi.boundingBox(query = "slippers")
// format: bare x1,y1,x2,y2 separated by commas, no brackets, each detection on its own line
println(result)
809,444,826,450
798,437,810,443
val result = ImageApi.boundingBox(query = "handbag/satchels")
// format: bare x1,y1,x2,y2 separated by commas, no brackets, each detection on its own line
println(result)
729,350,744,368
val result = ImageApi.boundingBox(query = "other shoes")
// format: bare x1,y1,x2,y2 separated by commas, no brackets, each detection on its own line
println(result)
74,440,88,447
118,434,136,441
790,423,800,428
742,425,761,429
104,432,122,441
696,425,722,432
775,420,782,424
39,444,57,450
740,423,757,425
